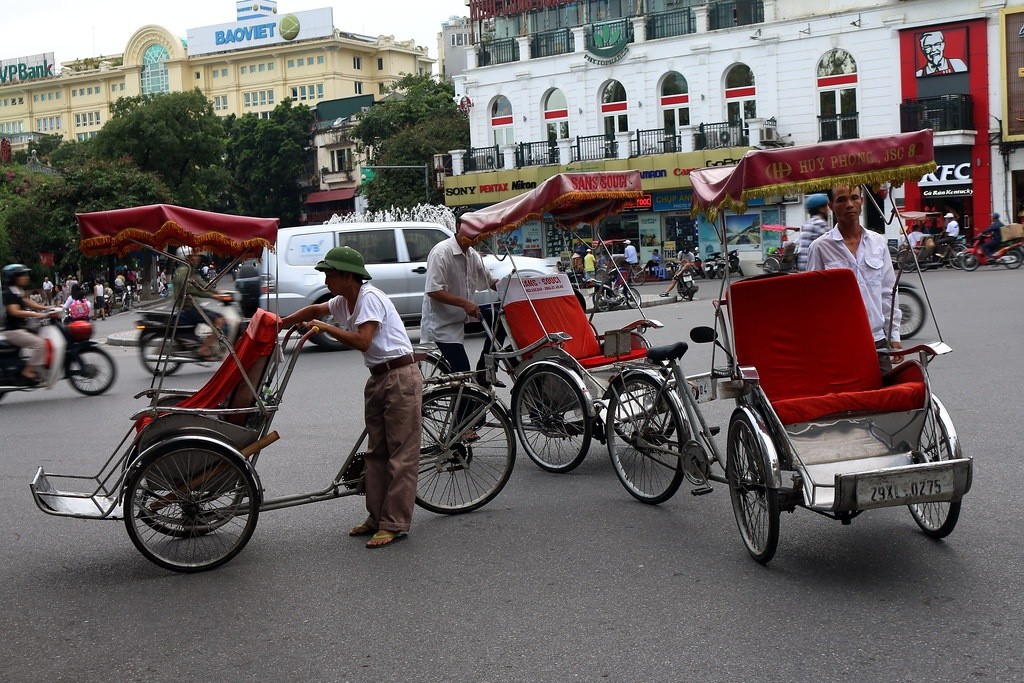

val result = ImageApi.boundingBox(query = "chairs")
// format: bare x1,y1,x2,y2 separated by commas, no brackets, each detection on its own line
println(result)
648,256,661,275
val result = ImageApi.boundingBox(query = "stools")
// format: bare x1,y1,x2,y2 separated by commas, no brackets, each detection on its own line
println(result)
659,266,670,279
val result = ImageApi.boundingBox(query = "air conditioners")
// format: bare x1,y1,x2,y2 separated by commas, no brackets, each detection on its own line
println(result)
718,126,741,146
759,128,777,141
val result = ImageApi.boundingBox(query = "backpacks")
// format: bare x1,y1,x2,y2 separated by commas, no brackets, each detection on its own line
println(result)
69,299,89,319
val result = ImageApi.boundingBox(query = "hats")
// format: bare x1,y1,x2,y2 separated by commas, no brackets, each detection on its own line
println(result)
239,264,242,266
944,213,954,217
623,239,631,244
571,253,581,259
315,245,372,280
586,249,592,252
989,213,1000,219
682,246,690,254
68,275,72,278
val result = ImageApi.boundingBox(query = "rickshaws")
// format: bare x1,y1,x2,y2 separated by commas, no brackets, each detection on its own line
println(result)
391,169,678,473
600,127,985,559
882,208,941,273
756,222,799,276
29,204,517,575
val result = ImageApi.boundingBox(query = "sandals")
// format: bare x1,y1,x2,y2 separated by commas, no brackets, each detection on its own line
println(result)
493,379,507,388
450,433,480,441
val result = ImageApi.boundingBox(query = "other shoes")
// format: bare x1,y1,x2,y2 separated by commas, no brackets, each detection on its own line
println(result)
102,317,106,320
672,275,679,280
935,253,944,258
659,291,669,296
985,258,994,261
19,373,38,380
91,318,97,320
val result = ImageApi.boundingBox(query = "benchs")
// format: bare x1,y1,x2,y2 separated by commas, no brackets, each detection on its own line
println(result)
494,273,664,372
725,269,953,425
129,308,280,441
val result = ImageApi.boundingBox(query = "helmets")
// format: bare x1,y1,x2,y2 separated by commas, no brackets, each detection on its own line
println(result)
210,265,214,270
176,246,194,259
805,194,828,213
2,264,32,284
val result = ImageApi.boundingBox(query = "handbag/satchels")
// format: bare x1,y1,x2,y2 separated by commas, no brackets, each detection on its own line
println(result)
915,240,921,247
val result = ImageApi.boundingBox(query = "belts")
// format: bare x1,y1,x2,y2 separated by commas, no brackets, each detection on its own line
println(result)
368,352,429,376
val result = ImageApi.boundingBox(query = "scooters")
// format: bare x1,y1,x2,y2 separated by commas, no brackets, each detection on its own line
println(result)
1,308,116,407
686,245,705,277
720,248,741,278
48,281,170,314
703,249,722,279
963,233,1023,273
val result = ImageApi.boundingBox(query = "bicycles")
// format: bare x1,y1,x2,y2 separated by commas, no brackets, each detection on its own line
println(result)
609,257,647,285
928,237,969,267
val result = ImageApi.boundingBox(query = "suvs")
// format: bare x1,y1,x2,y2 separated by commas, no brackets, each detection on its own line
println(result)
261,215,554,351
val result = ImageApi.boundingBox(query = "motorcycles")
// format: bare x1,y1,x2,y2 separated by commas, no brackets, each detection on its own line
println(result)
136,285,256,380
670,261,698,298
583,267,642,308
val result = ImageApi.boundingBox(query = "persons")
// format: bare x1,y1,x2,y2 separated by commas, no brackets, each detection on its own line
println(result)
1,263,59,385
173,247,229,361
931,207,945,232
644,249,660,275
806,182,906,379
596,252,606,266
32,257,218,327
924,206,931,234
417,207,505,441
278,246,427,548
556,261,563,273
659,245,696,297
618,239,639,269
946,205,959,230
907,225,924,249
935,213,959,258
979,213,1005,262
797,194,833,273
584,249,598,279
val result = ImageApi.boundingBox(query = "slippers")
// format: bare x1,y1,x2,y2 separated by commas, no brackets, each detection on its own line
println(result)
348,524,379,536
366,534,407,548
